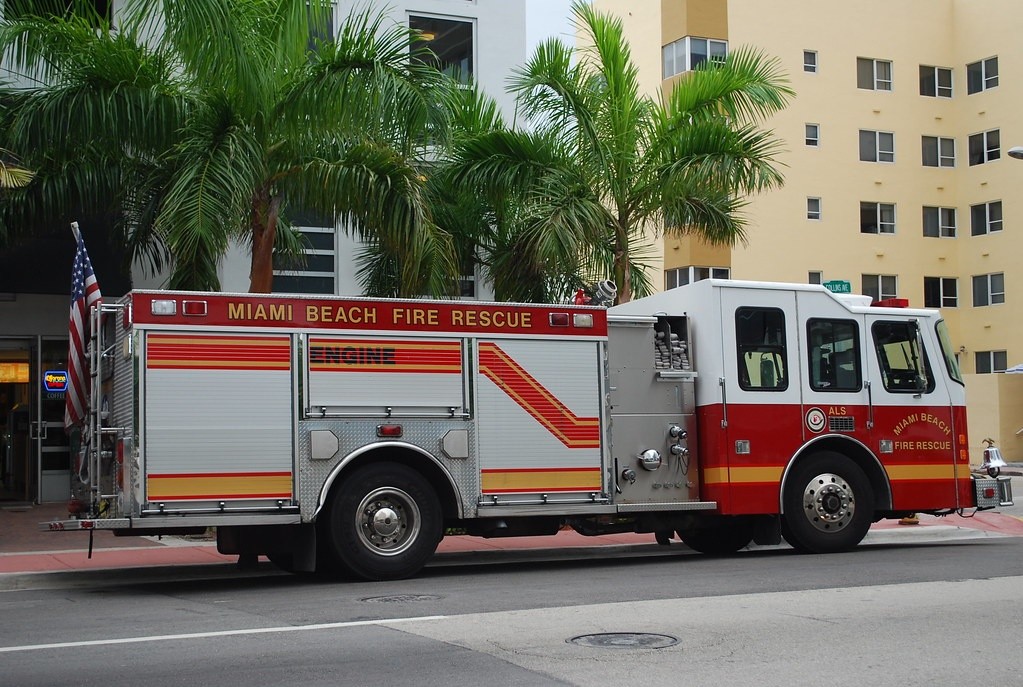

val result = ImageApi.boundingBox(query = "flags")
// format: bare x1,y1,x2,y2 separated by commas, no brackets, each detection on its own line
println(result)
63,221,109,428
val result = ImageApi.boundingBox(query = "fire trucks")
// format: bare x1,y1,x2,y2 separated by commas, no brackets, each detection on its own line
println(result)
41,277,1017,583
